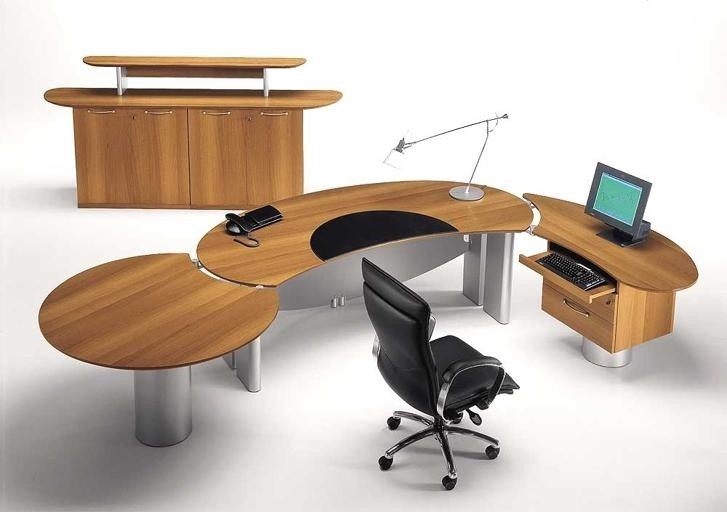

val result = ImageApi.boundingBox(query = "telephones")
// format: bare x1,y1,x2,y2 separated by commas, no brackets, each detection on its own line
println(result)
225,205,284,233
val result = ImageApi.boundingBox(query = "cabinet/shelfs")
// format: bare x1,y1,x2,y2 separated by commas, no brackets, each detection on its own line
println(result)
43,56,342,211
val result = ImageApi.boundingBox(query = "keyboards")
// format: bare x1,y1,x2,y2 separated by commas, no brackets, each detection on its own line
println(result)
535,252,608,291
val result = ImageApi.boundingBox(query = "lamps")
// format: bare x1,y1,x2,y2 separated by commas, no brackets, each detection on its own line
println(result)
383,110,510,202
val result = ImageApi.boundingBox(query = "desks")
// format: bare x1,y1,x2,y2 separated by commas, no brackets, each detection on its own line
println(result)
39,252,279,448
196,180,534,392
520,188,699,369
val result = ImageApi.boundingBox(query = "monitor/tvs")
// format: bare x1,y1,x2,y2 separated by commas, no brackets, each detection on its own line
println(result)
583,162,652,248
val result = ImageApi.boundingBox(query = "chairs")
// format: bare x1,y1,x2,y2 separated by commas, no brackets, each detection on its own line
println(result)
361,256,520,491
